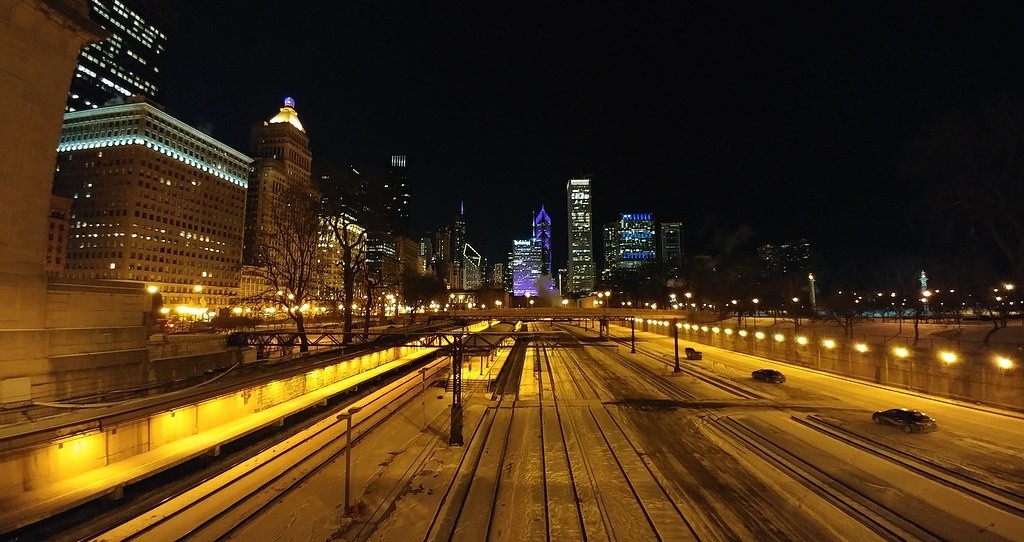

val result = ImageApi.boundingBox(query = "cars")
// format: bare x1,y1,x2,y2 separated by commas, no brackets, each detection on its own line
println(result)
872,407,937,434
752,369,787,384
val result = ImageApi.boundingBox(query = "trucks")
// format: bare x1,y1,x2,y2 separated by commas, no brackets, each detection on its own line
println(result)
685,348,703,360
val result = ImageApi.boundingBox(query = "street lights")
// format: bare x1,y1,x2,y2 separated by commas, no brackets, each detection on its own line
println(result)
336,406,364,509
417,366,430,430
